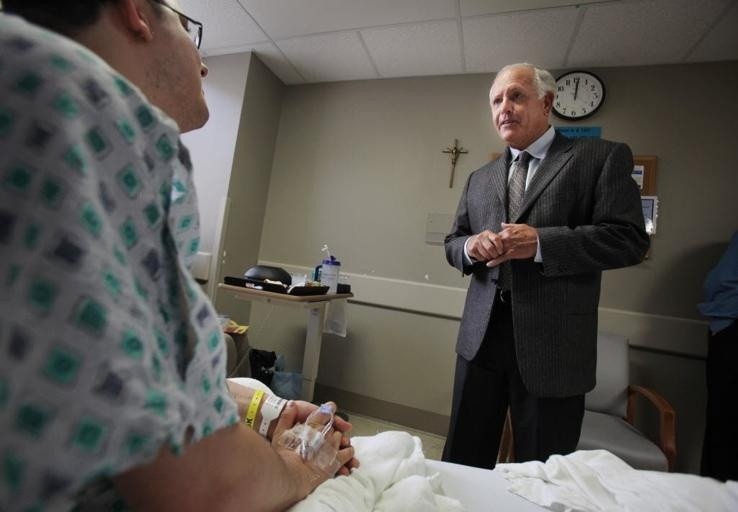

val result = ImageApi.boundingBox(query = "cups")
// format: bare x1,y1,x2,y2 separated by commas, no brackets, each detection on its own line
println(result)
314,255,342,294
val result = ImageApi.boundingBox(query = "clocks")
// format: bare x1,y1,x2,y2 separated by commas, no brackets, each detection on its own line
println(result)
549,70,606,121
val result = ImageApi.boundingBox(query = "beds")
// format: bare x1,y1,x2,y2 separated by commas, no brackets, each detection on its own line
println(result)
277,454,738,512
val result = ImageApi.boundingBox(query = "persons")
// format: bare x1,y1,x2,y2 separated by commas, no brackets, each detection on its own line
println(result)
0,0,360,512
440,62,652,470
696,229,737,483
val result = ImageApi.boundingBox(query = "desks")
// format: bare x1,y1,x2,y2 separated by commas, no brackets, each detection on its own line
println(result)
216,282,353,405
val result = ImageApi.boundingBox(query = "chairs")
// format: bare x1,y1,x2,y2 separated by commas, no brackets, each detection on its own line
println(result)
496,327,679,472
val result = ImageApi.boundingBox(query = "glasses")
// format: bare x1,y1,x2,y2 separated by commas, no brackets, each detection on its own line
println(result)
159,1,202,49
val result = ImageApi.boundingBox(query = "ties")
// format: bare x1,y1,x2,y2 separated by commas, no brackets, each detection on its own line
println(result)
499,152,530,291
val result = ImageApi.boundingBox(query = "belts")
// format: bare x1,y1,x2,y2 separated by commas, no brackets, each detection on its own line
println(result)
493,287,512,303
